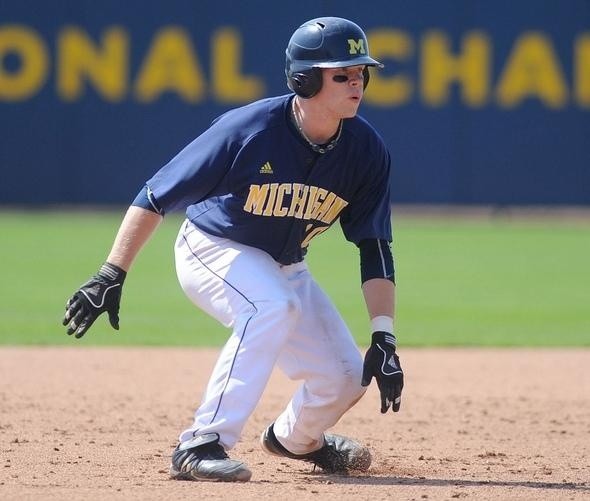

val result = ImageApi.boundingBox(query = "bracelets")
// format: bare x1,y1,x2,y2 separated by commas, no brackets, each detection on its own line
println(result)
371,315,394,334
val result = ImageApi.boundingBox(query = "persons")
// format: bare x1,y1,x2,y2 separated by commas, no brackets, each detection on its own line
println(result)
64,16,403,480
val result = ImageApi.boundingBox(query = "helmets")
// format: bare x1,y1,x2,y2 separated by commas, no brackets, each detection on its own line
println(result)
284,16,386,100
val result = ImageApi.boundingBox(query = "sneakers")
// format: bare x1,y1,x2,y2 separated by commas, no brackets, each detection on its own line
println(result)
261,422,372,473
168,431,252,482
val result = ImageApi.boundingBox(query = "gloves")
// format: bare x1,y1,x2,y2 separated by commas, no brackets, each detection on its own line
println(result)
360,331,404,414
60,262,127,339
